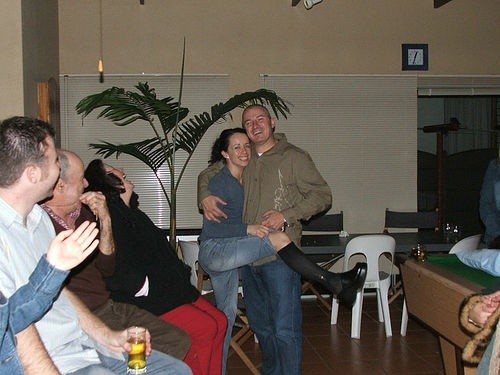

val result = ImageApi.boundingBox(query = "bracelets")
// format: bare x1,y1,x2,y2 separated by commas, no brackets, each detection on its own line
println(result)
284,218,288,227
468,319,484,329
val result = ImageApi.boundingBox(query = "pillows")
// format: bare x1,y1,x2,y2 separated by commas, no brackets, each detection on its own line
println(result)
448,233,500,278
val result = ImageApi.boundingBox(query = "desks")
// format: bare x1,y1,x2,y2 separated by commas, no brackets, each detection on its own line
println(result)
301,234,500,375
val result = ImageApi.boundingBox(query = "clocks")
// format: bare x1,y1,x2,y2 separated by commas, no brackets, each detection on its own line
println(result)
402,44,428,71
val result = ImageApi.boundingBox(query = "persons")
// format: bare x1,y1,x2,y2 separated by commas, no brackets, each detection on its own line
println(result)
467,289,500,375
0,116,193,375
479,157,500,250
82,159,228,375
197,104,332,375
199,128,367,375
37,149,191,360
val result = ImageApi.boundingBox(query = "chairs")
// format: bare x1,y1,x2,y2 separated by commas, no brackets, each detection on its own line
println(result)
197,209,438,375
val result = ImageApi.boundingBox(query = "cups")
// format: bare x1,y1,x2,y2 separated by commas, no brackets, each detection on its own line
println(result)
127,326,147,374
443,222,461,243
412,242,425,263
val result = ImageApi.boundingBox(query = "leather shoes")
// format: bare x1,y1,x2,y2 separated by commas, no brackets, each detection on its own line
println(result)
337,262,368,308
